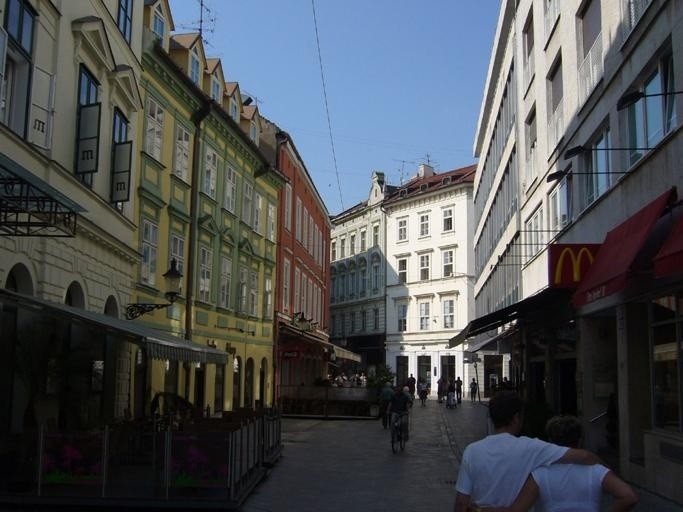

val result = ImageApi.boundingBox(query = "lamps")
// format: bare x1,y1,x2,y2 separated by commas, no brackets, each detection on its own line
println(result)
544,90,682,184
124,256,184,320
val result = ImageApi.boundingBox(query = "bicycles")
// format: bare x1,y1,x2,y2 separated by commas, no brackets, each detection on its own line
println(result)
388,408,409,454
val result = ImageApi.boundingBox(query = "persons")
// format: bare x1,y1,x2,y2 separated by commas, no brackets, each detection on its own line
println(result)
468,412,640,511
453,389,602,511
380,382,393,432
328,367,508,410
386,385,413,442
